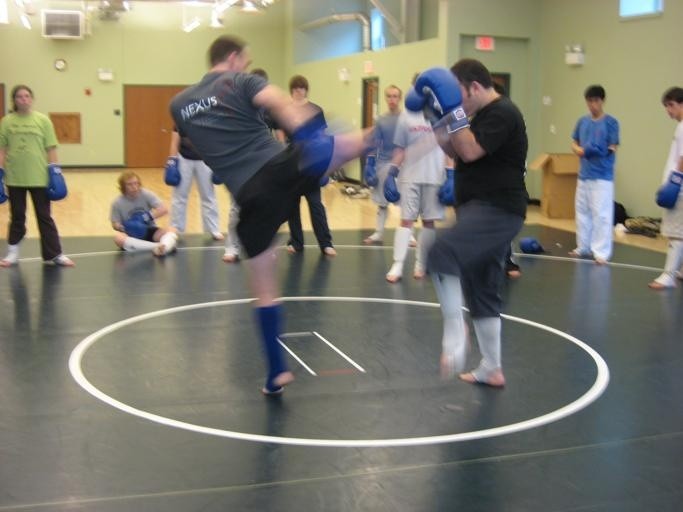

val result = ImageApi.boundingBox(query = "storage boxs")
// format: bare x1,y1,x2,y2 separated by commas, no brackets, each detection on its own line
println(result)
528,152,580,219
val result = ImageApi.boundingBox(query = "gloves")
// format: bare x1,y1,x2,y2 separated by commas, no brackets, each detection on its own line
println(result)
0,168,8,204
414,67,471,134
124,220,149,239
164,156,182,186
583,140,609,161
128,210,153,225
437,166,457,206
363,155,379,187
404,88,446,129
45,164,68,201
518,236,544,254
655,169,683,208
211,171,224,186
382,164,401,202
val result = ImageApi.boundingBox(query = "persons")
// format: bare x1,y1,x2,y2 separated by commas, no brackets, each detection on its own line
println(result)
109,171,179,256
219,68,284,263
169,33,375,395
382,72,454,285
163,121,224,241
0,85,75,267
648,86,683,290
568,84,620,265
364,85,418,247
286,75,337,256
403,58,530,389
